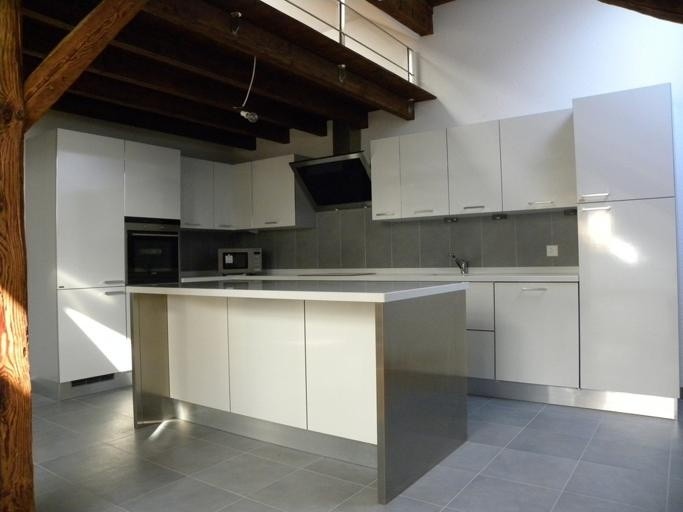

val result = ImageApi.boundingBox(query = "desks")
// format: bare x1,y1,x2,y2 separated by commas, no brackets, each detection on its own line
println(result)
124,279,465,506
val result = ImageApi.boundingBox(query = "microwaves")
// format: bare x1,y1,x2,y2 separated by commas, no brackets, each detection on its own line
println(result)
216,247,263,274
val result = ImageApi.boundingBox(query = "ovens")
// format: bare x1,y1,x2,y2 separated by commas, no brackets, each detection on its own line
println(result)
122,219,181,286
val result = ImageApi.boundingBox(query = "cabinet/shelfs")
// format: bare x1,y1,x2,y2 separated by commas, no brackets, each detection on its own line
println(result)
217,160,253,229
466,283,495,397
371,136,400,221
571,82,677,205
399,126,450,220
124,139,181,221
25,127,127,290
27,287,128,401
447,119,505,220
253,152,317,229
180,152,216,232
577,195,681,420
493,281,579,407
499,108,577,217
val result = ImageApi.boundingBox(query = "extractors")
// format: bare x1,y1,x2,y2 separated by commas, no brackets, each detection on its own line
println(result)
286,116,372,213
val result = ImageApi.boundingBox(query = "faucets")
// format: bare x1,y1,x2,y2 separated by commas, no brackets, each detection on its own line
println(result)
452,254,468,274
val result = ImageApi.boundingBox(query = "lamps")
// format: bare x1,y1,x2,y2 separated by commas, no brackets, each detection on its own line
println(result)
231,56,260,125
